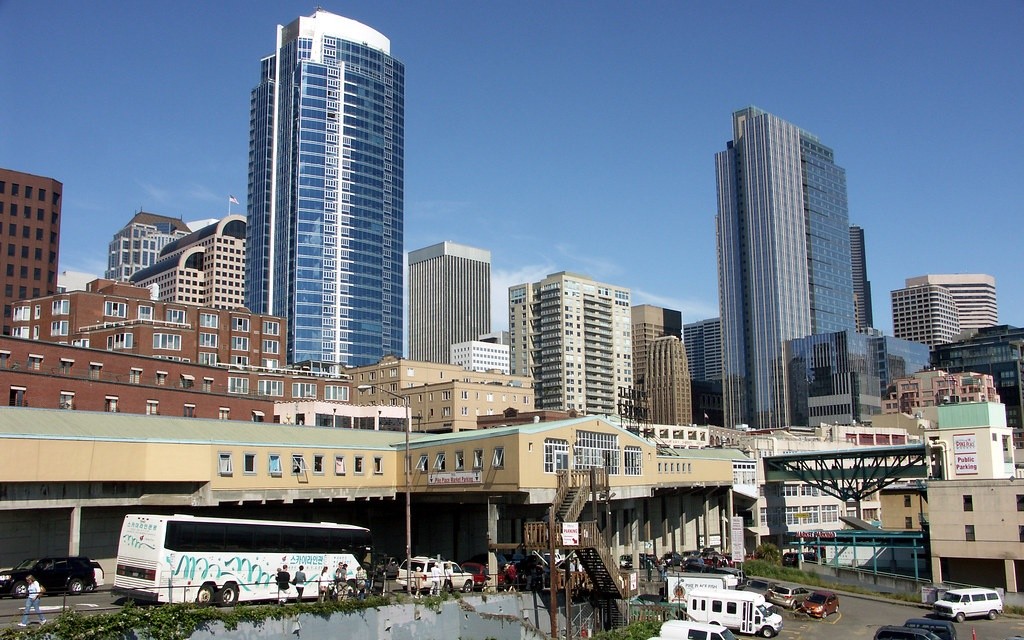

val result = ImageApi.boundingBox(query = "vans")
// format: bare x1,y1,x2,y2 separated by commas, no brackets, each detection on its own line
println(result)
782,552,818,568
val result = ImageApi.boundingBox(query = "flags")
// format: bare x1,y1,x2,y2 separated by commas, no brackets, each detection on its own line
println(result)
229,193,239,204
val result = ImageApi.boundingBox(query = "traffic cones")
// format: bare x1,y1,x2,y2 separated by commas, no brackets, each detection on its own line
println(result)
972,627,977,640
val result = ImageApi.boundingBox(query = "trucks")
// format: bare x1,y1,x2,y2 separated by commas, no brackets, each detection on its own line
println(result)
664,573,748,606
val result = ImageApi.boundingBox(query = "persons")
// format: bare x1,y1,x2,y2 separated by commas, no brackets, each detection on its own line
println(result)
503,558,584,593
443,563,453,594
646,559,667,581
276,565,290,606
17,575,47,627
483,563,490,594
414,567,424,599
295,565,306,604
711,554,718,572
698,556,705,573
336,561,382,602
320,566,328,603
430,562,441,597
722,556,729,567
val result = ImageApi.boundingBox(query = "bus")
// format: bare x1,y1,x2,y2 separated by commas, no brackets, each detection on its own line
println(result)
686,588,783,638
109,512,377,609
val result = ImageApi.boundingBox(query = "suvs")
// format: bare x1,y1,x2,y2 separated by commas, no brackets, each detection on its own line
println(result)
85,559,105,593
933,588,1003,623
0,555,97,599
460,562,505,588
658,619,742,640
395,556,474,595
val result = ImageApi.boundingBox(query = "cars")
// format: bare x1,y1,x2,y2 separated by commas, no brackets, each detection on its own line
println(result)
802,590,839,619
903,618,958,640
874,624,943,640
767,584,812,610
742,579,776,599
620,548,755,571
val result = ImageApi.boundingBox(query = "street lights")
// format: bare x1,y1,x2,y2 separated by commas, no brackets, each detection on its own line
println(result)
357,384,412,594
